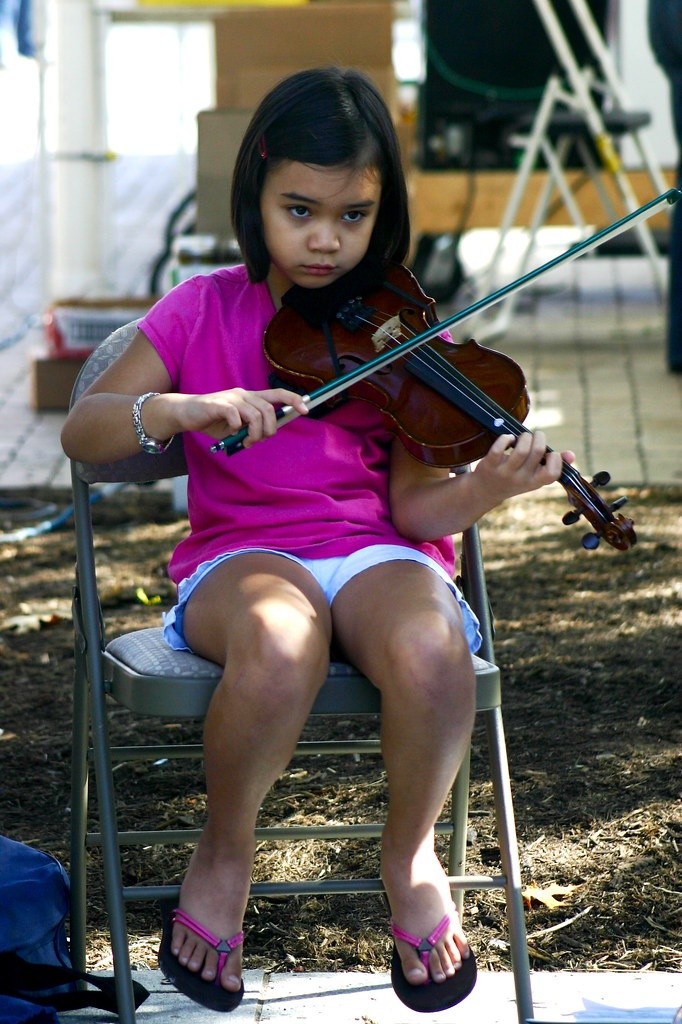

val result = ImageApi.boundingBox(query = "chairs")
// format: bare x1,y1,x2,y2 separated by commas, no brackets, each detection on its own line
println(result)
66,319,535,1022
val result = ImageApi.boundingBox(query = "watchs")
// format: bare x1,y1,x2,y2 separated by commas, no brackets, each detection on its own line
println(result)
132,390,175,455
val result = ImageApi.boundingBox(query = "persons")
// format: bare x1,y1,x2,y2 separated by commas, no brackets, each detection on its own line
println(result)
645,0,682,376
62,67,578,1017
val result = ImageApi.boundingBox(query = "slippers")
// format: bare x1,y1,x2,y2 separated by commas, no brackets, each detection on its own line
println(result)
157,896,247,1012
391,900,478,1012
